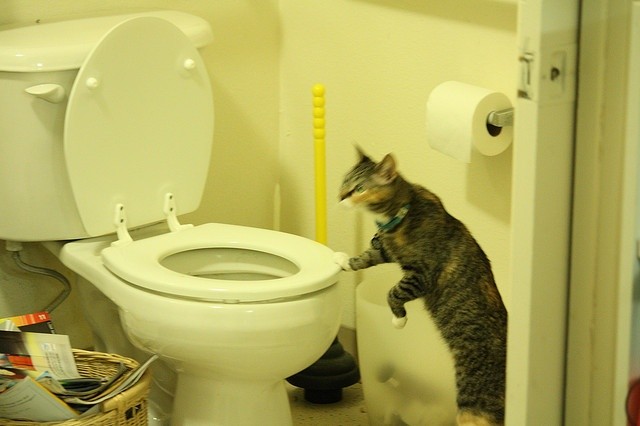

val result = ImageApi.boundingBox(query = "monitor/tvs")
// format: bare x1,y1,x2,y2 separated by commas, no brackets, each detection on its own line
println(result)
0,310,161,423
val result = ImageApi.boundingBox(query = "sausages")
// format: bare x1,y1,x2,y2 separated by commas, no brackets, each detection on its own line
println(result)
332,139,508,426
